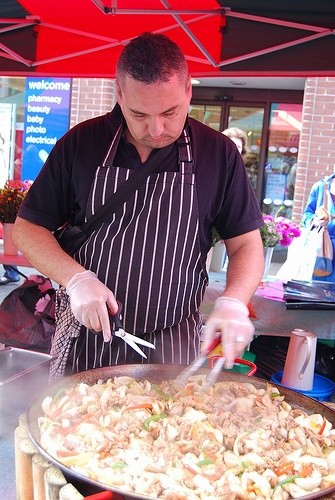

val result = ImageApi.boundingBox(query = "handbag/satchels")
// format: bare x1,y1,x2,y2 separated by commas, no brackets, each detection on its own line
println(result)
275,217,324,283
310,178,334,276
53,216,90,258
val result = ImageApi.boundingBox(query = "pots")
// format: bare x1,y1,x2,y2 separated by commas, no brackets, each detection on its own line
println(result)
27,357,335,500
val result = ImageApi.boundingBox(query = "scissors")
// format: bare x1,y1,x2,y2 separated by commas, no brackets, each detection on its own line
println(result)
96,301,155,360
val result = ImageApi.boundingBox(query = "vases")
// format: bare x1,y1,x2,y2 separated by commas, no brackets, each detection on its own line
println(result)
262,247,274,280
210,240,227,273
3,224,18,256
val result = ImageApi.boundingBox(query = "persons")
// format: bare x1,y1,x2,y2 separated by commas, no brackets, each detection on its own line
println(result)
12,31,265,381
221,127,247,157
302,164,335,288
244,151,259,191
282,156,297,202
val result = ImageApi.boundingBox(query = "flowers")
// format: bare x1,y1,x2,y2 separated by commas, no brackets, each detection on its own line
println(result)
261,212,303,247
0,180,34,224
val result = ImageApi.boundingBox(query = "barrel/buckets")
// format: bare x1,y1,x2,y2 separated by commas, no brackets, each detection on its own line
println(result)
271,370,335,402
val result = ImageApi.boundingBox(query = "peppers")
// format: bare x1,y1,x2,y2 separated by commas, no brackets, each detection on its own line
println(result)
47,383,326,494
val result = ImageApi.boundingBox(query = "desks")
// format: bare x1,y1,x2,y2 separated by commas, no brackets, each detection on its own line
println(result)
0,238,33,268
200,274,335,350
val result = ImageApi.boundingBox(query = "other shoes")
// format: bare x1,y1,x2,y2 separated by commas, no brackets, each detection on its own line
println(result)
0,276,19,284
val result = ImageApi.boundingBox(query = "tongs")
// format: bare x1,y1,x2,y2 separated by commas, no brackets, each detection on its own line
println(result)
172,334,225,398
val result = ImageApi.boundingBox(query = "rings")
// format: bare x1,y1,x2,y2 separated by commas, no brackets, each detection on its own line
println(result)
235,335,245,343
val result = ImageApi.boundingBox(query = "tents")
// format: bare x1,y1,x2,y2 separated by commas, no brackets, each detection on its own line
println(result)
209,108,301,155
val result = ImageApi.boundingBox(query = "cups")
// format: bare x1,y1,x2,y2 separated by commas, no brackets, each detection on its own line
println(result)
281,328,318,391
